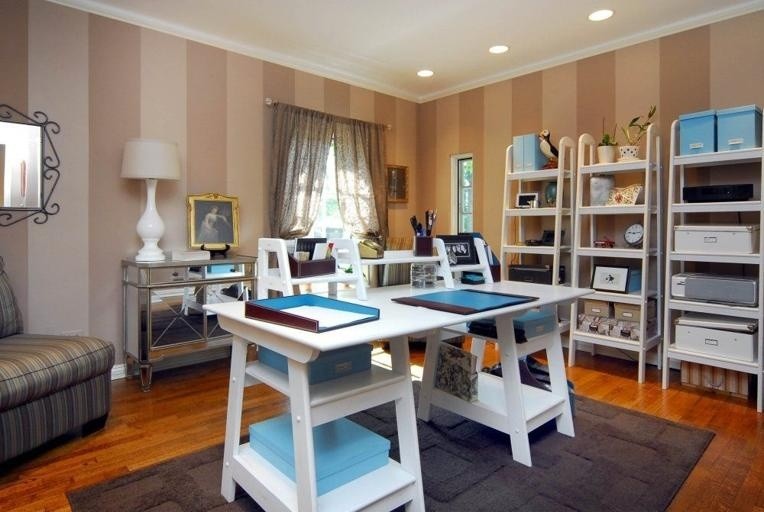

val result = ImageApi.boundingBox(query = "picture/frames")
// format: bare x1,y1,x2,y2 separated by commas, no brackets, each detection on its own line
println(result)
185,192,240,251
384,164,409,203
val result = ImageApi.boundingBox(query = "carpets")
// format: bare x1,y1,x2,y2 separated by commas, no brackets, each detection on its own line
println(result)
63,378,717,512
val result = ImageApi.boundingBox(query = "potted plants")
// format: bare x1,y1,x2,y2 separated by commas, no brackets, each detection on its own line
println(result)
617,105,657,160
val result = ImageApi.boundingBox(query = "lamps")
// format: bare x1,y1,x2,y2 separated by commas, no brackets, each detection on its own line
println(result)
120,138,183,264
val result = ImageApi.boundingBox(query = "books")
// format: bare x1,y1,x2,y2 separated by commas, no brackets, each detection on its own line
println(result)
293,237,329,261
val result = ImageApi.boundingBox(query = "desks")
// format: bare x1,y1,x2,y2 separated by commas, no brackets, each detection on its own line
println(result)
201,280,597,512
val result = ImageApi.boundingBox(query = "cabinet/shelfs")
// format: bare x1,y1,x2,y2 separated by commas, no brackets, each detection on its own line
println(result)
182,264,249,318
120,253,257,392
256,237,495,301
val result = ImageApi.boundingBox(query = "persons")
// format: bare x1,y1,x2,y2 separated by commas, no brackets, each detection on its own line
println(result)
388,169,403,199
198,203,231,243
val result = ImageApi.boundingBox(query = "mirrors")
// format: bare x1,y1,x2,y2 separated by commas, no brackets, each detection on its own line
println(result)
0,103,61,228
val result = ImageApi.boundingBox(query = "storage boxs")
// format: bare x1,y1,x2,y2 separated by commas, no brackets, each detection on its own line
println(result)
677,104,762,154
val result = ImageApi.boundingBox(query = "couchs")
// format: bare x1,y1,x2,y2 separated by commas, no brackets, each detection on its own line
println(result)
0,255,117,472
